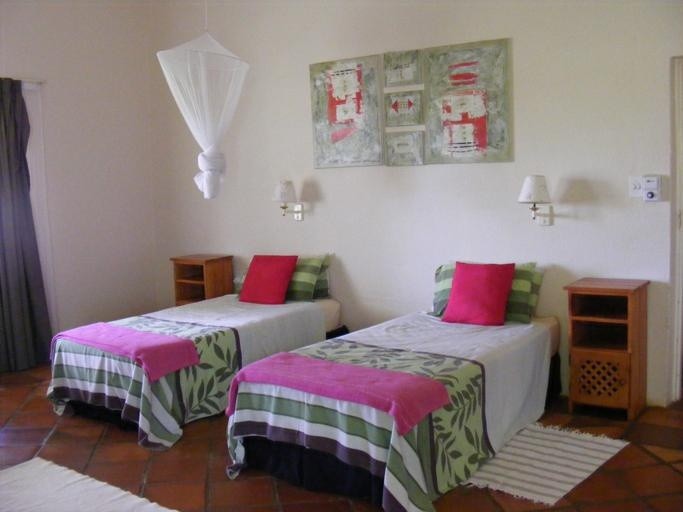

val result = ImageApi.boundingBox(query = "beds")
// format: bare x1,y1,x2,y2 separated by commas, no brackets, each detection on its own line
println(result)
219,299,561,510
46,296,340,450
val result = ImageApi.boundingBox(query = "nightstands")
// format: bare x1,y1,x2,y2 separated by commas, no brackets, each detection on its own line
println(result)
167,252,232,305
563,273,650,421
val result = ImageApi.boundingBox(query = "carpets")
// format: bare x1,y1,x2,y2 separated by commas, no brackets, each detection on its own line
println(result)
0,455,184,511
460,423,628,506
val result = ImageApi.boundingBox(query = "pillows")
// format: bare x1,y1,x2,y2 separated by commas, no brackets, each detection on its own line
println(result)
240,254,297,304
530,268,542,318
285,254,326,304
430,261,538,325
442,260,516,329
315,254,333,297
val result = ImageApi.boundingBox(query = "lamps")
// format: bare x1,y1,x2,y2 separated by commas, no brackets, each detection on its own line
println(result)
269,177,303,223
518,173,557,227
